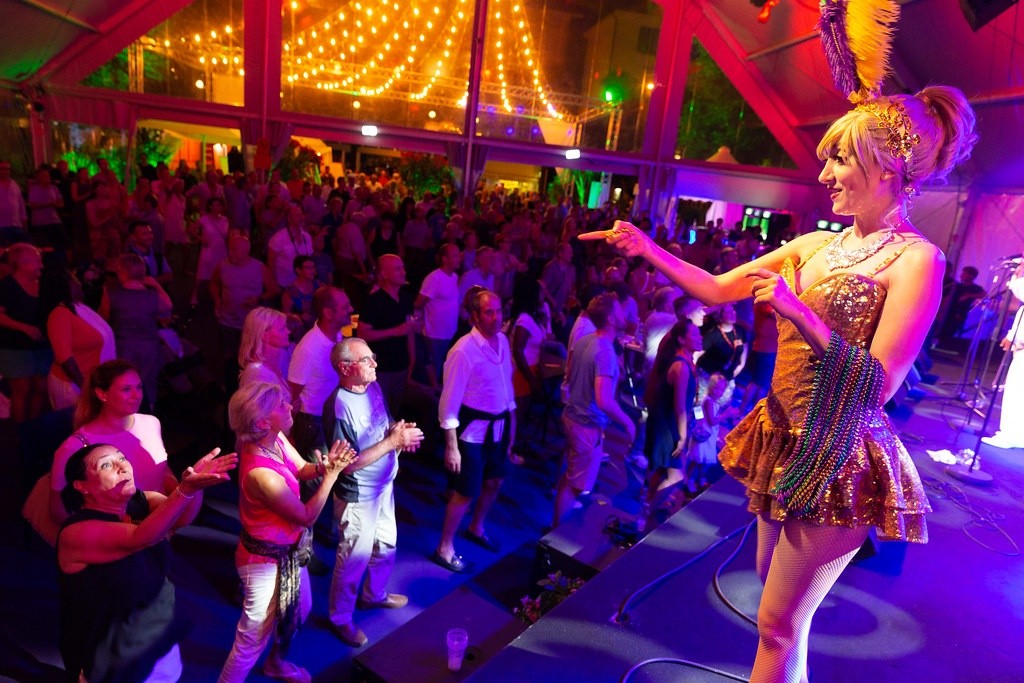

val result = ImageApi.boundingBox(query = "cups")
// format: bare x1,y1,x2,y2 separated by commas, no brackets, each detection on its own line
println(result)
447,628,468,671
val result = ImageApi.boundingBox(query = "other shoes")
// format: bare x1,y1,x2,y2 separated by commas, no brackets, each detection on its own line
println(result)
357,592,409,610
981,437,1013,450
510,453,525,467
308,556,330,577
463,527,501,553
432,549,476,575
313,529,340,548
333,621,368,647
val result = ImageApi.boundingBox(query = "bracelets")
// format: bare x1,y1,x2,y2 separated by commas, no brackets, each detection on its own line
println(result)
176,487,194,499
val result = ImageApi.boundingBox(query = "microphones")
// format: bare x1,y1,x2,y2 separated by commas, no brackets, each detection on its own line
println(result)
998,254,1024,269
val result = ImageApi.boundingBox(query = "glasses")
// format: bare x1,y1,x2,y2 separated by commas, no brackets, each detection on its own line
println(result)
343,354,377,365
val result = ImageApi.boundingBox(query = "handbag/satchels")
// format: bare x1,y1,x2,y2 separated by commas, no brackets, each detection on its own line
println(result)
22,433,89,547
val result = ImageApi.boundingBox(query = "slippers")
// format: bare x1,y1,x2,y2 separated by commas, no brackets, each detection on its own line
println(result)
264,662,311,680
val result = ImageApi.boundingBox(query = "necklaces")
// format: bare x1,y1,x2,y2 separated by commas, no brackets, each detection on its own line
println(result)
769,331,885,518
824,214,910,271
254,441,298,480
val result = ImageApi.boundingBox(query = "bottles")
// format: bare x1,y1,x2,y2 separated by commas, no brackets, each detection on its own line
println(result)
969,401,983,408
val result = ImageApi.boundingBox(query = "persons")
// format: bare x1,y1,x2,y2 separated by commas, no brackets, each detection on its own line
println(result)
980,250,1024,449
926,260,986,339
577,85,977,683
0,137,802,683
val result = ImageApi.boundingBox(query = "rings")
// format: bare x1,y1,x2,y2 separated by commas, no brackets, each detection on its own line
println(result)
341,458,348,463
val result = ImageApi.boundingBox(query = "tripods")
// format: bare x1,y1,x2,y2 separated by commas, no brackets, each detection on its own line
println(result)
925,264,1019,418
